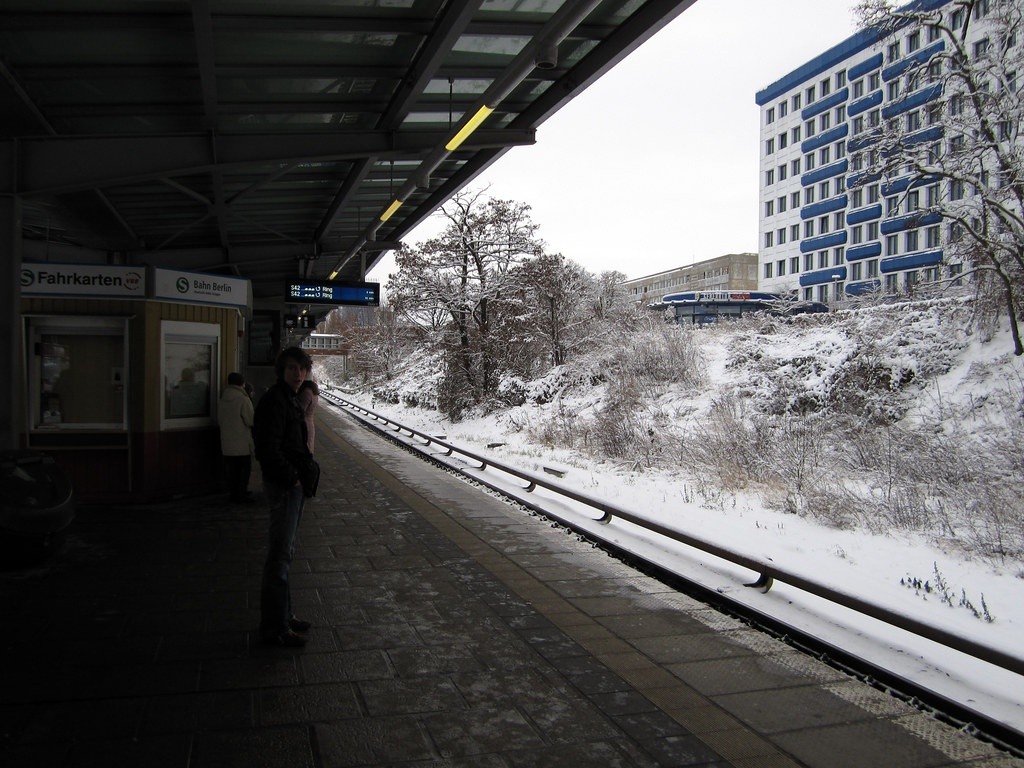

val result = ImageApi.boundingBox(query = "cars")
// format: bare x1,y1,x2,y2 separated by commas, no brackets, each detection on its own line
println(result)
790,300,829,315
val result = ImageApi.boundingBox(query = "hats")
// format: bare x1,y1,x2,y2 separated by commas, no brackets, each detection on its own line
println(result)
228,372,245,385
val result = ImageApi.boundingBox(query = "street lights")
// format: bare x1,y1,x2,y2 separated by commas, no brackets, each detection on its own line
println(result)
832,275,841,313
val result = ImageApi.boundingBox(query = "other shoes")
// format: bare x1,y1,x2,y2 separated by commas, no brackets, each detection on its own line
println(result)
257,623,309,648
306,495,320,503
288,606,311,633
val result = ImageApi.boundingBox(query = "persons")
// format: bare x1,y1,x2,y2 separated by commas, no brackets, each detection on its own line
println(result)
217,372,319,502
253,348,319,650
